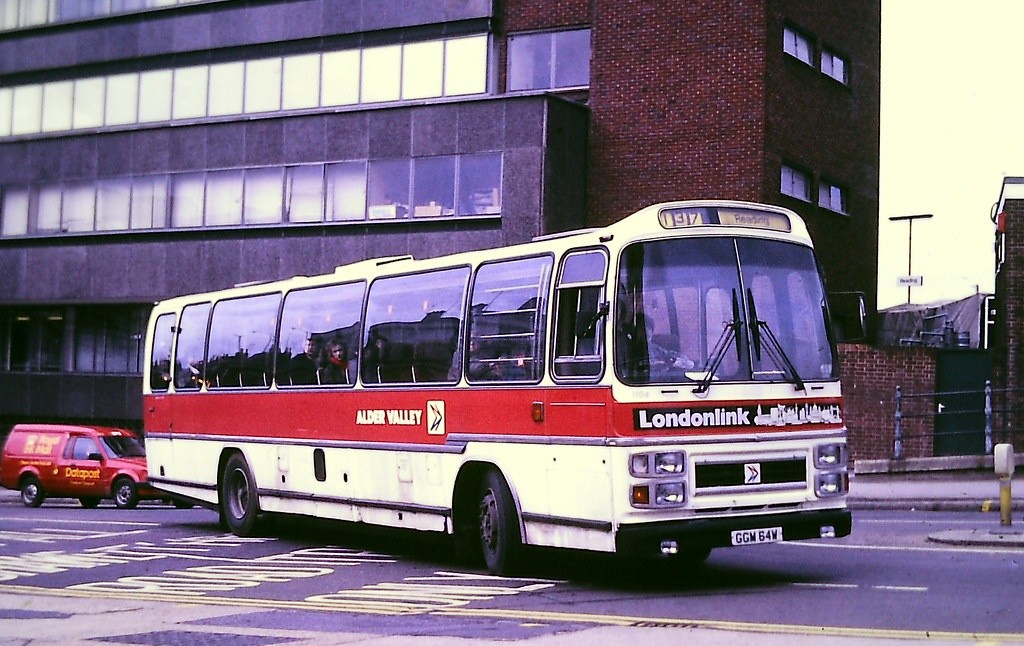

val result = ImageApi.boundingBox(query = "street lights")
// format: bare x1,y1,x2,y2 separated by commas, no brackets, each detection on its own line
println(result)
889,211,936,305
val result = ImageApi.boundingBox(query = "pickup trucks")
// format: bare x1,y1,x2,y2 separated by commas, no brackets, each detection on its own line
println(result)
0,424,193,510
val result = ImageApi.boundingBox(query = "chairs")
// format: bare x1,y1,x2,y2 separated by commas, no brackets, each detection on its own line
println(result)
150,338,525,390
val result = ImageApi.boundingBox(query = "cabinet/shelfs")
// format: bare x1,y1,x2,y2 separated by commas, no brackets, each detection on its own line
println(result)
369,205,396,219
413,204,441,217
473,188,498,207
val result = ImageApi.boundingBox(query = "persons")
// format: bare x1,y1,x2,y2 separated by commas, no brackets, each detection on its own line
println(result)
448,298,693,383
83,442,98,459
150,335,390,390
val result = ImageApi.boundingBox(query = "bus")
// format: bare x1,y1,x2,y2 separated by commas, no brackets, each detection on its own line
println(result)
144,198,854,574
